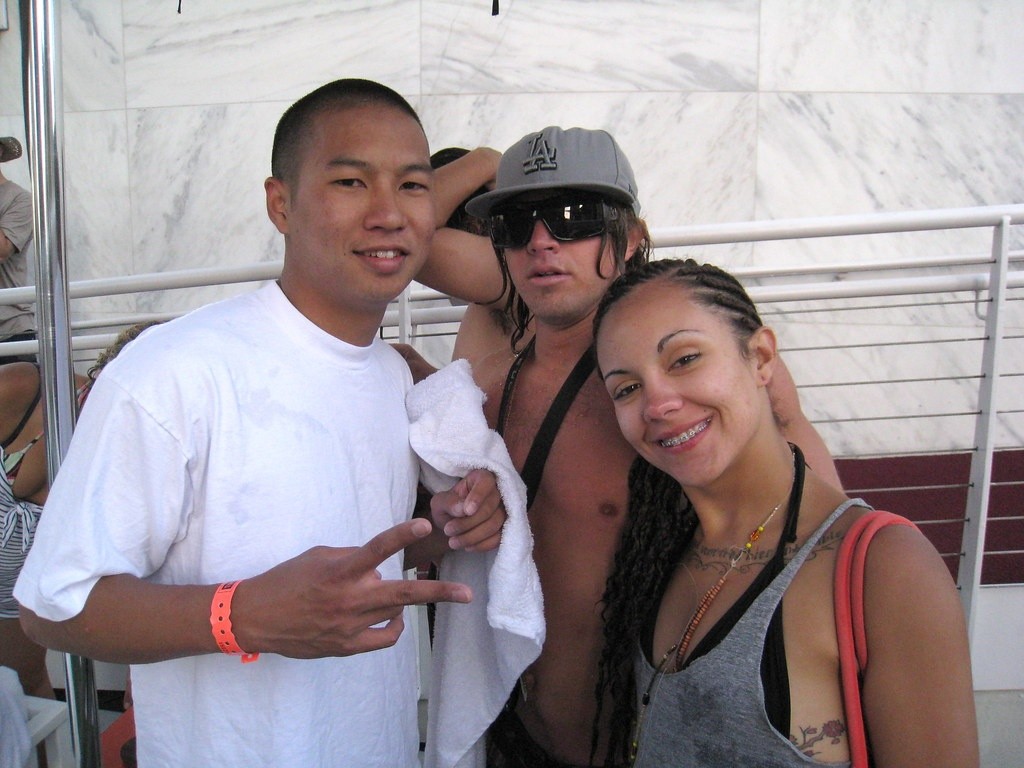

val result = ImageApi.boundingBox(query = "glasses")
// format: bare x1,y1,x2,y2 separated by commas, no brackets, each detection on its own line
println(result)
487,193,618,248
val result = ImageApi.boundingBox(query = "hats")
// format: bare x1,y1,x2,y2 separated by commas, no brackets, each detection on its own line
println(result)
465,126,642,221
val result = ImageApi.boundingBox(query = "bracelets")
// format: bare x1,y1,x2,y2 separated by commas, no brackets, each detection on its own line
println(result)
210,579,260,664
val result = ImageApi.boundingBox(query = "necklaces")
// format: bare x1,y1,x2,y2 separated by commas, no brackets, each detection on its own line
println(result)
625,450,798,766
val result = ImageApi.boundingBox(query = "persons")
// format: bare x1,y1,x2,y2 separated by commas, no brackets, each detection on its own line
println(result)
12,78,508,768
412,144,535,369
597,257,980,768
0,134,36,365
0,319,167,768
389,126,851,768
